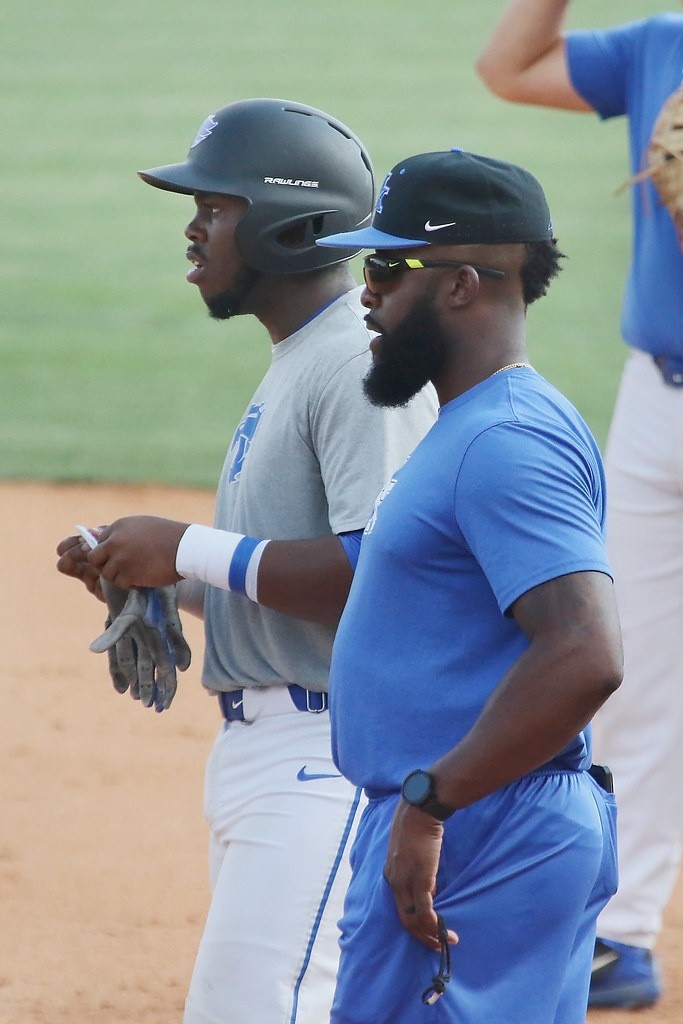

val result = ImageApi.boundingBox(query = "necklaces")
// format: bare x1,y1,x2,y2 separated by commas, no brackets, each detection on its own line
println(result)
496,363,530,373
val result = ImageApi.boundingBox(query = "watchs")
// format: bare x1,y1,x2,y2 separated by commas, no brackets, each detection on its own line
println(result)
402,769,456,822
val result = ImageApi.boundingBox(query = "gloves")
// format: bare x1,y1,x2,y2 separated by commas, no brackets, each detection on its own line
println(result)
89,543,193,712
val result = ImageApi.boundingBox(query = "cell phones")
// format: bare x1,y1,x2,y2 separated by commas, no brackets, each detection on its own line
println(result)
586,764,615,794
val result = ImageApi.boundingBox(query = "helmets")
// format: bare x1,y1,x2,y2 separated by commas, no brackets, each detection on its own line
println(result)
137,97,378,276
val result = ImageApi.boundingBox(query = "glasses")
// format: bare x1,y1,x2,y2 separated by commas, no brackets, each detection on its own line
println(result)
363,254,506,294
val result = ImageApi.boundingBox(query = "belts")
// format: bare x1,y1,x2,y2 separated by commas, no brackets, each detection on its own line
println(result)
217,683,332,723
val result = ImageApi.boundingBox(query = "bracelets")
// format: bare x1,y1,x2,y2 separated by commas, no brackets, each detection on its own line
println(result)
175,522,270,606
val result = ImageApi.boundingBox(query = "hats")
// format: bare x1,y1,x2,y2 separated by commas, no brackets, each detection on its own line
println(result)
315,150,553,250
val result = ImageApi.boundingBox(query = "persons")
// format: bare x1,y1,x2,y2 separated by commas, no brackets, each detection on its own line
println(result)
52,98,438,1024
312,150,627,1024
476,1,683,1010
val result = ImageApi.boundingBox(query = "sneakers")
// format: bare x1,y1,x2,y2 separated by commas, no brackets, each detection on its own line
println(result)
586,940,658,1009
